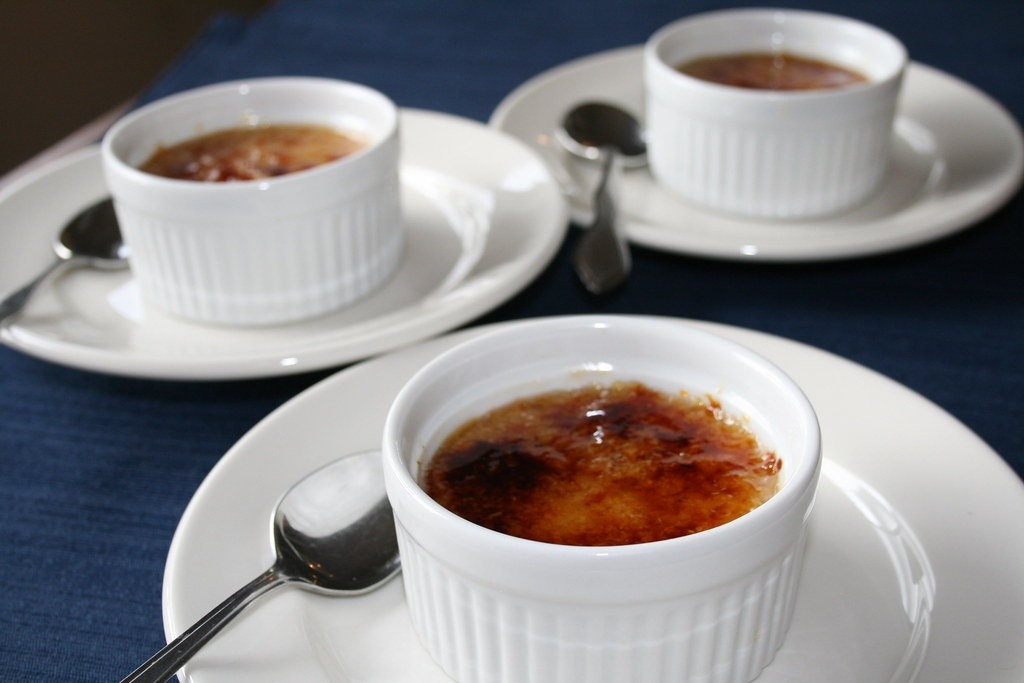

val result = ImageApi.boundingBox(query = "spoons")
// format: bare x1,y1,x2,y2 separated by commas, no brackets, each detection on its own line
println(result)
0,197,131,327
115,451,405,683
558,98,652,294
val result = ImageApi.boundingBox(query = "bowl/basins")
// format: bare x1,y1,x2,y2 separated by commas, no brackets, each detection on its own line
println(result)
644,9,909,224
103,76,403,327
381,317,823,683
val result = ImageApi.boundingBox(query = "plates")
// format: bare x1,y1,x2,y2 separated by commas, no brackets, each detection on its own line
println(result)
0,105,570,381
484,45,1023,263
158,319,1023,683
